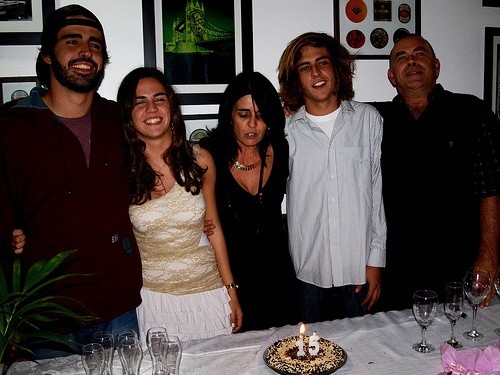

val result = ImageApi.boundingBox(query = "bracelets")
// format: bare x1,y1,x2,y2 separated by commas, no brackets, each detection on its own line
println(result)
225,283,238,289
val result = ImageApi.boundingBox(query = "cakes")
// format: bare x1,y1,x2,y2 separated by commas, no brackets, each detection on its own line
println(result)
265,334,344,374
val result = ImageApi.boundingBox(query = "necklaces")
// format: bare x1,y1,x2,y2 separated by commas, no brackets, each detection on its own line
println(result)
232,159,261,171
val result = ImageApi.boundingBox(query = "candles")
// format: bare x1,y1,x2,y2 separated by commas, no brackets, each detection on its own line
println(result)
298,323,305,355
308,330,320,355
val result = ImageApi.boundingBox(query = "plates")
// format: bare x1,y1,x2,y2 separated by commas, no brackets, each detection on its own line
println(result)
262,345,348,375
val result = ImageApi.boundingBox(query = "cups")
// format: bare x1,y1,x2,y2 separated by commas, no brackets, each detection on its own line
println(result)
159,335,182,375
81,328,143,375
146,327,168,375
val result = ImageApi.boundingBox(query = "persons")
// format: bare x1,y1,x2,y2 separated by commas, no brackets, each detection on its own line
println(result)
10,67,243,350
276,31,388,324
372,35,500,313
198,72,299,333
0,4,144,361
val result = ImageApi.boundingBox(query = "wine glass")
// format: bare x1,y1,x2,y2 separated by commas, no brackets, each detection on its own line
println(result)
492,268,500,336
443,281,465,348
412,290,437,353
463,267,491,341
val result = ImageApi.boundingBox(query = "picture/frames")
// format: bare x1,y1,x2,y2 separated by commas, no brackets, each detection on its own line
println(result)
141,0,254,121
0,0,56,46
333,0,422,60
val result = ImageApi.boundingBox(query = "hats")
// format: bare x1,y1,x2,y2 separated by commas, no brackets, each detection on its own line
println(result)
36,4,105,88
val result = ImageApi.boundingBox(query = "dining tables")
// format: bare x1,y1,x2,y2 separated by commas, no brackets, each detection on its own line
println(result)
6,290,500,374
